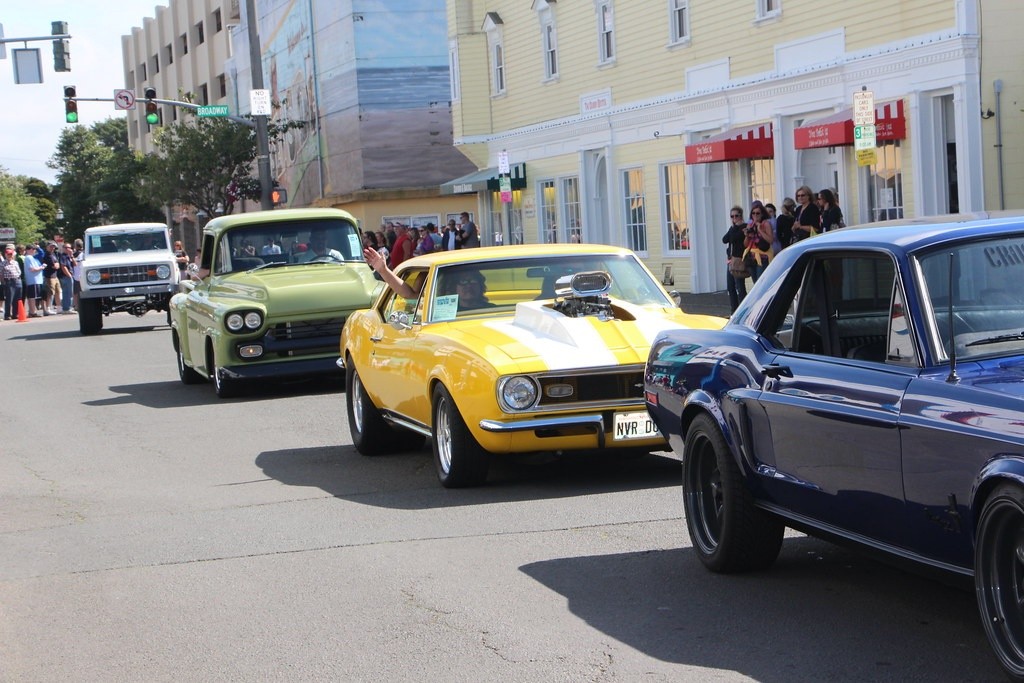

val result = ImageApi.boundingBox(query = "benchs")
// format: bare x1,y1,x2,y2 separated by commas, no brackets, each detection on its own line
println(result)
799,303,1024,361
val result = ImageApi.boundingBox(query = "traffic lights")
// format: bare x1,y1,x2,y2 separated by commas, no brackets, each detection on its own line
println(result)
144,87,158,124
63,85,78,123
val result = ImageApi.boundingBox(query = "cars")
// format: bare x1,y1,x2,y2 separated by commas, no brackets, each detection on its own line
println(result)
169,207,387,399
340,243,731,490
642,212,1024,683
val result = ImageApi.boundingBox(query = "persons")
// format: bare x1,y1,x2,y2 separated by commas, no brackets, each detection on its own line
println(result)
240,237,308,258
300,226,344,263
174,240,213,282
358,211,480,281
0,237,85,320
723,184,847,317
364,246,494,313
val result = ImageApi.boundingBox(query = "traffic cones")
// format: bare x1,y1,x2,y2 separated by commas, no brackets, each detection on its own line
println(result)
16,300,30,322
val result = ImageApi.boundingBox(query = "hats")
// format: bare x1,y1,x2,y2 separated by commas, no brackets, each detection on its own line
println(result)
6,244,15,249
5,249,13,255
391,221,404,226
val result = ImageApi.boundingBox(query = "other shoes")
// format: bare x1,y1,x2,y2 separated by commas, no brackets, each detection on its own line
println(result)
62,310,77,314
43,310,62,315
28,312,42,318
5,316,18,320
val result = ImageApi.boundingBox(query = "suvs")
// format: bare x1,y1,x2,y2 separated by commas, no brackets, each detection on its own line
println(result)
78,222,181,333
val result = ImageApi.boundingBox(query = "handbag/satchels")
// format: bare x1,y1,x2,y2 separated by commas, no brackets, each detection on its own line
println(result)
789,230,810,244
729,257,751,278
755,238,770,252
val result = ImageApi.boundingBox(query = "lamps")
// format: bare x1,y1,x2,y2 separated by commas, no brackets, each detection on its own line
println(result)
653,131,682,138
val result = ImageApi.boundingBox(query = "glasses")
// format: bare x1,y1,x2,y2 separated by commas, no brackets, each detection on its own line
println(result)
751,211,762,215
460,216,464,219
457,278,479,285
817,198,823,200
730,215,741,218
64,247,71,249
797,194,805,197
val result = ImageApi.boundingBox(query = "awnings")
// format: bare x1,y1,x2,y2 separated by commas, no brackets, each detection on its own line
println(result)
794,98,906,150
685,121,775,165
440,161,528,195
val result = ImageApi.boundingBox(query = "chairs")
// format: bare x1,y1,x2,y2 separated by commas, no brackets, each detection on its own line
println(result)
289,252,306,264
531,271,565,300
232,257,265,270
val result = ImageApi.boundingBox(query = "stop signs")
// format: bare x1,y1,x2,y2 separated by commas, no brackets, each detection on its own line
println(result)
272,189,288,204
